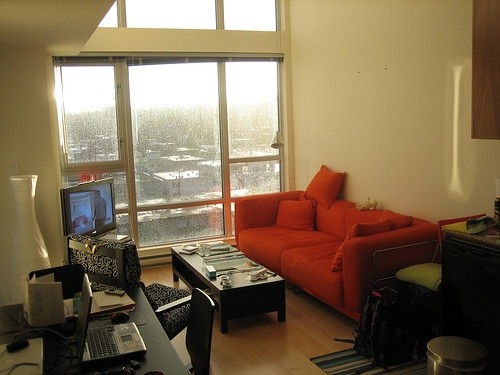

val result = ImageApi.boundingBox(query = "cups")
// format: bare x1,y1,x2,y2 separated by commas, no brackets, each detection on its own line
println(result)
202,244,211,258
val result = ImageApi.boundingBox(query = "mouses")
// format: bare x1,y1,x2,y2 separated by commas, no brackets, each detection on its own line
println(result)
111,311,129,322
6,338,29,352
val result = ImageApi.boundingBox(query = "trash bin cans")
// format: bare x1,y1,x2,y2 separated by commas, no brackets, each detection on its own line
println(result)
426,336,488,375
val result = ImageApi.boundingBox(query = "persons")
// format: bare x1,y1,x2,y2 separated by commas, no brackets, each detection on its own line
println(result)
93,190,107,229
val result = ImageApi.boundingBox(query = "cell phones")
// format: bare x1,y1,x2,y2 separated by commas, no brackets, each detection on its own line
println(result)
105,288,126,296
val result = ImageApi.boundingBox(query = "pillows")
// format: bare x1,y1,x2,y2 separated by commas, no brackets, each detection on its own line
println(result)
331,215,414,271
276,199,316,231
304,165,346,209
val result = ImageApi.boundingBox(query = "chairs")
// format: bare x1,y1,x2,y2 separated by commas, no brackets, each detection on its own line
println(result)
395,242,442,295
63,234,192,341
185,288,215,375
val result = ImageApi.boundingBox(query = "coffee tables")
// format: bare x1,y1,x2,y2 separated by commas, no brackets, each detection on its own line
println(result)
171,241,286,334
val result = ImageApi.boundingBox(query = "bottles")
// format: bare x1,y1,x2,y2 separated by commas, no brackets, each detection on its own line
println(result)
10,174,54,321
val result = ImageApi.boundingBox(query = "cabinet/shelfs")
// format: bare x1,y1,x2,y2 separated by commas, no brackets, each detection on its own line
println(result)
440,222,500,375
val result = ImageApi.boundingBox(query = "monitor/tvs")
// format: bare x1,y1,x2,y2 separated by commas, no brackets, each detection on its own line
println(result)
59,177,117,238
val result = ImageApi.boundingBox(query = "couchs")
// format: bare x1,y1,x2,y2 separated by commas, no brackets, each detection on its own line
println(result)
235,191,438,323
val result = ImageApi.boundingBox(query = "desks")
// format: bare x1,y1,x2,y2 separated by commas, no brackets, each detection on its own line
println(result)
43,287,188,375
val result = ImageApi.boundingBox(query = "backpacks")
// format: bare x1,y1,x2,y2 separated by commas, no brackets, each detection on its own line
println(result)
352,287,413,366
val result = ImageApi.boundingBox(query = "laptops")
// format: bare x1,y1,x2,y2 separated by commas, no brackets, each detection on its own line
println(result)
76,273,147,364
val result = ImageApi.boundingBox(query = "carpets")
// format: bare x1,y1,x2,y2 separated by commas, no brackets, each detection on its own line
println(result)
309,348,429,375
